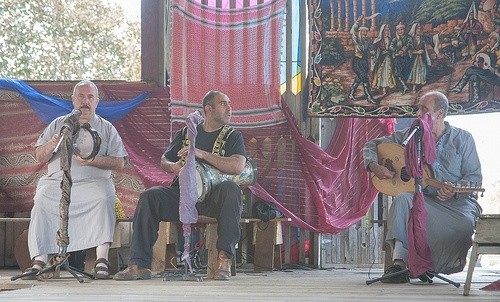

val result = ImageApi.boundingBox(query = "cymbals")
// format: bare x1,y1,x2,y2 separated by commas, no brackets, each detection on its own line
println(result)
71,124,103,159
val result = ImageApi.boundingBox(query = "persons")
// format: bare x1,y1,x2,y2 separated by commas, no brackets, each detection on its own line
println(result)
363,92,482,287
112,89,248,281
21,80,129,280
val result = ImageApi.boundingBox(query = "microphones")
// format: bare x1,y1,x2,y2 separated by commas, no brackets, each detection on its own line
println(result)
72,109,82,117
186,110,201,123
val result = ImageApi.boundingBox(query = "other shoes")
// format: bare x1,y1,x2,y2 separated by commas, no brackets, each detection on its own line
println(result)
381,266,410,283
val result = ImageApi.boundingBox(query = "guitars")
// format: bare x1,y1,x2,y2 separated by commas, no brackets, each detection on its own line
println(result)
371,143,487,199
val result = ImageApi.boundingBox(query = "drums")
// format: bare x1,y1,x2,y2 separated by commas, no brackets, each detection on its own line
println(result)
195,156,257,204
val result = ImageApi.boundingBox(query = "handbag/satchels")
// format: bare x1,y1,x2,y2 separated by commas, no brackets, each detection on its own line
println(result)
255,200,283,230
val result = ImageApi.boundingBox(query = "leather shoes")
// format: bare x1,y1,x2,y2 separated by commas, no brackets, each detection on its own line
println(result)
214,251,232,280
113,265,151,281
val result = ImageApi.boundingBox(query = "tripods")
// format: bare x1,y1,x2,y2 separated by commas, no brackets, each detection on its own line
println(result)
10,136,95,284
366,126,460,288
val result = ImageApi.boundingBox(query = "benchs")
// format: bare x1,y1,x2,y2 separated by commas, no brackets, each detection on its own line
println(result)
463,215,500,297
0,212,292,277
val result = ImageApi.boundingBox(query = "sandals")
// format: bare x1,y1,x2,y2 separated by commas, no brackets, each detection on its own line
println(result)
21,260,46,280
94,258,111,279
